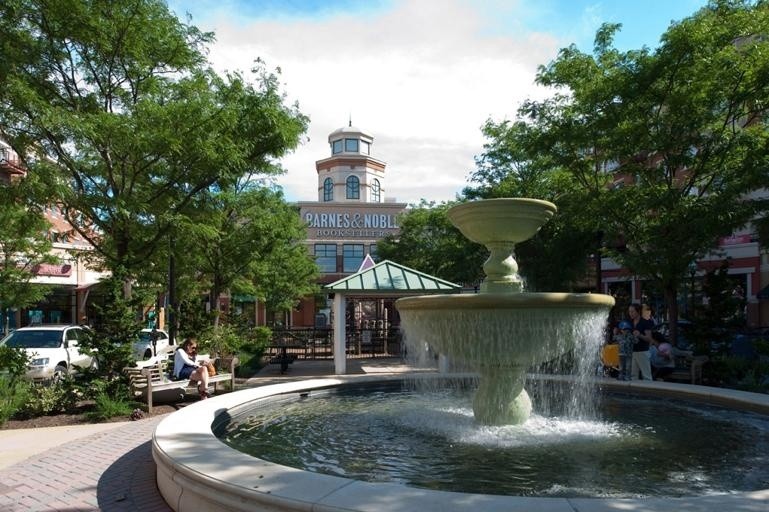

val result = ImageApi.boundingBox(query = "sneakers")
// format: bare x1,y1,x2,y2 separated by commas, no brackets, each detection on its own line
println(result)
200,389,211,397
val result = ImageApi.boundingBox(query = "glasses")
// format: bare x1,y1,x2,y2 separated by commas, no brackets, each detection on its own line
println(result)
191,346,196,350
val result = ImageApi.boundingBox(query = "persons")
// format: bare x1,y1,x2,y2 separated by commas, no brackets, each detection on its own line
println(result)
638,332,676,381
172,337,211,400
612,302,654,382
612,322,639,381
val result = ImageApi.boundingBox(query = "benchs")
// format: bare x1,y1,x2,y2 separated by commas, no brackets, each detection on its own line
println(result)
671,355,706,385
123,345,240,414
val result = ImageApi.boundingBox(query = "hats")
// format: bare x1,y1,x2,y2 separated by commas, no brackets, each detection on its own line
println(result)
619,322,632,330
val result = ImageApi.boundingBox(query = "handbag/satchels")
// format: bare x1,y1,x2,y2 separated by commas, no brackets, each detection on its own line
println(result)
203,362,216,376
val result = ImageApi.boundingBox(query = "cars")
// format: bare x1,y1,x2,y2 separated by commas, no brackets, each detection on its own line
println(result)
0,325,177,387
